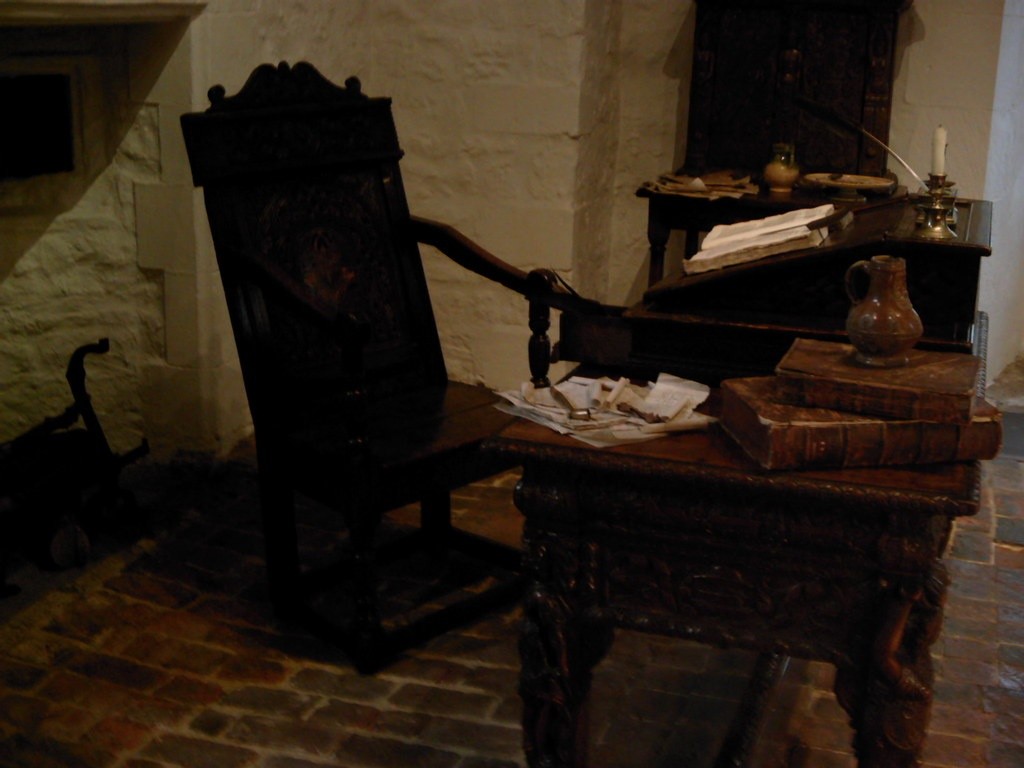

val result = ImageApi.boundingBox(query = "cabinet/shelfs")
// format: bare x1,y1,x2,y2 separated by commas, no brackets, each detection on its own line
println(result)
683,0,907,180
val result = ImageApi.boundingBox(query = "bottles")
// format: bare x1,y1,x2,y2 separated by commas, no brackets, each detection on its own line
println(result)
846,255,924,369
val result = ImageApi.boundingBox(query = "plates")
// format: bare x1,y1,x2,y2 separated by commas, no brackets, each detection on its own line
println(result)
804,173,894,189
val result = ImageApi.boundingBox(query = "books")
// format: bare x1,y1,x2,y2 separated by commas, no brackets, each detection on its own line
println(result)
683,204,835,276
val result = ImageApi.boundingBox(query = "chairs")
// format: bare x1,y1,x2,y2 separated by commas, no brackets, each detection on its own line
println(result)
180,61,622,676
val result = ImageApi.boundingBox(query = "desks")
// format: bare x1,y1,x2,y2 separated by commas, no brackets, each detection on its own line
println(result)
634,164,863,289
479,310,989,768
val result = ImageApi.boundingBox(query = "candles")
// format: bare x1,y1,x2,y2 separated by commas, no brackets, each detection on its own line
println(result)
930,124,949,177
